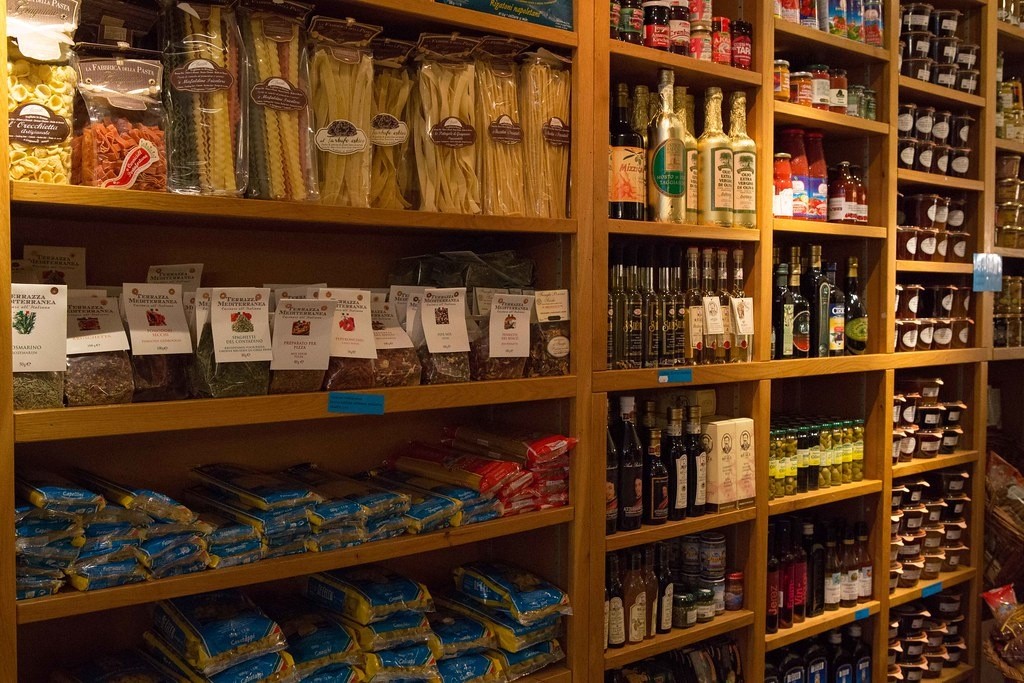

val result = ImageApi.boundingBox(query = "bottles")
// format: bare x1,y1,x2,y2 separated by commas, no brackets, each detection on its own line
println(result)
766,524,873,634
608,68,756,228
607,246,753,370
605,396,707,535
771,245,869,360
608,554,626,648
772,127,872,226
604,587,609,653
623,550,646,644
765,627,871,683
656,543,674,634
642,548,658,639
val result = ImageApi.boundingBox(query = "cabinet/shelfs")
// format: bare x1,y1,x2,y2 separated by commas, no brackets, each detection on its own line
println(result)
0,0,1024,683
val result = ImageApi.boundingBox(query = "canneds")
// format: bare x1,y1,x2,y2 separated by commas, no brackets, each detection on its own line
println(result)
610,0,752,70
998,0,1024,29
671,533,743,628
767,414,864,500
775,59,877,120
993,276,1024,347
995,156,1024,250
996,51,1024,143
